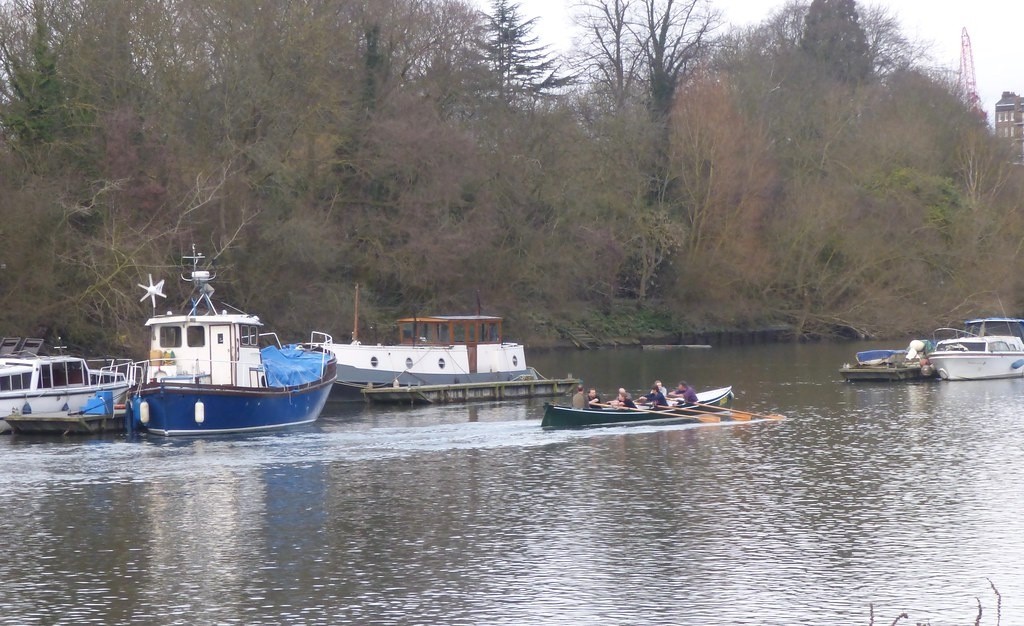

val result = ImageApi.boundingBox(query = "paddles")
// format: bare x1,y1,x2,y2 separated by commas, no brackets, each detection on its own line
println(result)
590,402,721,424
646,404,751,421
698,403,787,421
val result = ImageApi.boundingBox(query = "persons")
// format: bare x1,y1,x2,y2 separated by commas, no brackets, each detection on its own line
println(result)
606,388,637,412
572,384,589,409
586,388,601,408
639,379,669,411
668,380,699,408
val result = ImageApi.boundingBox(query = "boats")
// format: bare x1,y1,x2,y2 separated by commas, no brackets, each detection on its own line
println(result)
289,280,542,404
838,316,1024,381
122,243,339,437
0,334,144,436
540,385,736,431
359,378,584,407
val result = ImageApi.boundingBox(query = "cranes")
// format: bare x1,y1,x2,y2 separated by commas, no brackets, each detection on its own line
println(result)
954,27,990,122
